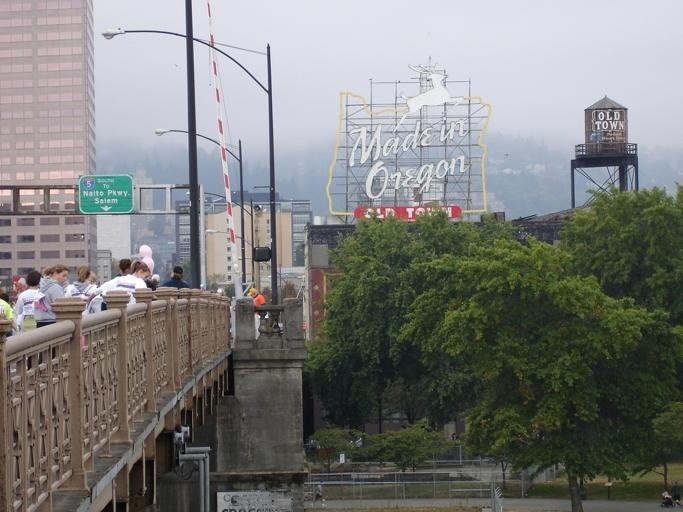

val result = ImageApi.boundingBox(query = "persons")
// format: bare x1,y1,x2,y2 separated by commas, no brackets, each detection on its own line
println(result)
310,478,327,509
0,258,227,380
660,480,680,509
246,286,265,338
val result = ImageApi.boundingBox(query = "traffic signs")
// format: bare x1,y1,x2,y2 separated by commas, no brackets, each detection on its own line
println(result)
78,174,134,216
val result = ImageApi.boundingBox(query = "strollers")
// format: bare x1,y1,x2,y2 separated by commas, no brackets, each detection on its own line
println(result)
660,491,676,508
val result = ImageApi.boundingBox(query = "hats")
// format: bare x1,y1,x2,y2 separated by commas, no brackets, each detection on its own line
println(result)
18,278,25,285
174,267,183,273
152,275,160,282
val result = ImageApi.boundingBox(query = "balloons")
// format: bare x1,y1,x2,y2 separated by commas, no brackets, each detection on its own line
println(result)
139,245,152,258
